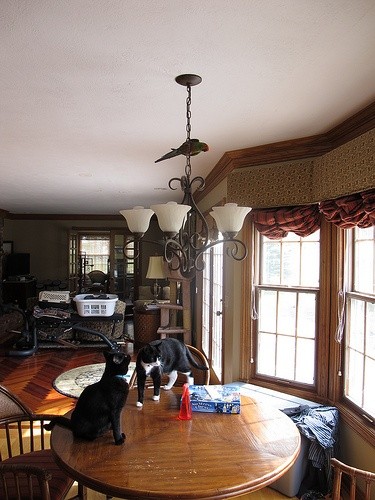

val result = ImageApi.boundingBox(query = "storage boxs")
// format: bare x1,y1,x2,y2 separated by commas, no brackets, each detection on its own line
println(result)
73,294,119,318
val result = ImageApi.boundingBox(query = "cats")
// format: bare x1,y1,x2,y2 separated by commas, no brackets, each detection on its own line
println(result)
42,349,131,446
135,338,209,407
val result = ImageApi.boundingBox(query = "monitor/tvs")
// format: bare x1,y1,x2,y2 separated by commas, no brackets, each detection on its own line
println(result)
2,252,31,280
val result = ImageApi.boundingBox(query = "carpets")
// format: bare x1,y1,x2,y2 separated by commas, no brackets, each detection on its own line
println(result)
53,361,137,400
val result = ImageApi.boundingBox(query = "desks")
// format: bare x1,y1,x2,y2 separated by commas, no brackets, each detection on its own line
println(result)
132,307,160,344
0,278,37,308
4,305,123,356
49,384,301,500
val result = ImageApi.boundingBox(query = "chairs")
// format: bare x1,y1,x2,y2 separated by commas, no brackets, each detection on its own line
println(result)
326,456,375,500
129,343,211,389
85,270,111,294
0,385,88,500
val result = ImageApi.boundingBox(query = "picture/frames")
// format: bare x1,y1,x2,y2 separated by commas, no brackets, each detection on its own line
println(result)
2,240,14,252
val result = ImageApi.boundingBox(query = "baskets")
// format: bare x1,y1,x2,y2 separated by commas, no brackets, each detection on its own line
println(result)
73,294,119,316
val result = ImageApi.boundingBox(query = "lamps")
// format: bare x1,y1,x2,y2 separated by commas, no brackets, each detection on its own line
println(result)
145,256,167,304
119,73,253,280
